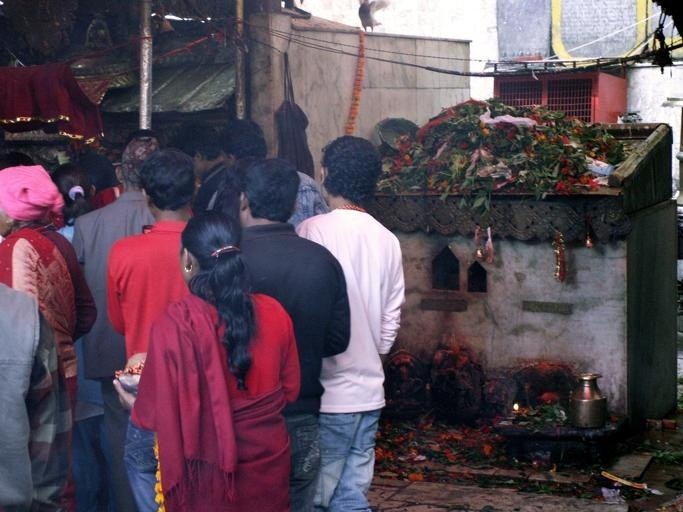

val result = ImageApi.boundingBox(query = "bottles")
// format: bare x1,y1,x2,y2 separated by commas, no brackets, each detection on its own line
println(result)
568,372,610,430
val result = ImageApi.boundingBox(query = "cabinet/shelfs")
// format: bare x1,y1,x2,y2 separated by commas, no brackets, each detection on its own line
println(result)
494,69,628,122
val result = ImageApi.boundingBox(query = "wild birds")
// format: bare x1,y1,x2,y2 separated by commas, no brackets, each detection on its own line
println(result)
358,0,391,34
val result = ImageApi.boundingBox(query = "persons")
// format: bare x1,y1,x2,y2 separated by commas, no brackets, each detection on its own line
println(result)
233,159,349,512
0,115,326,512
293,135,404,512
